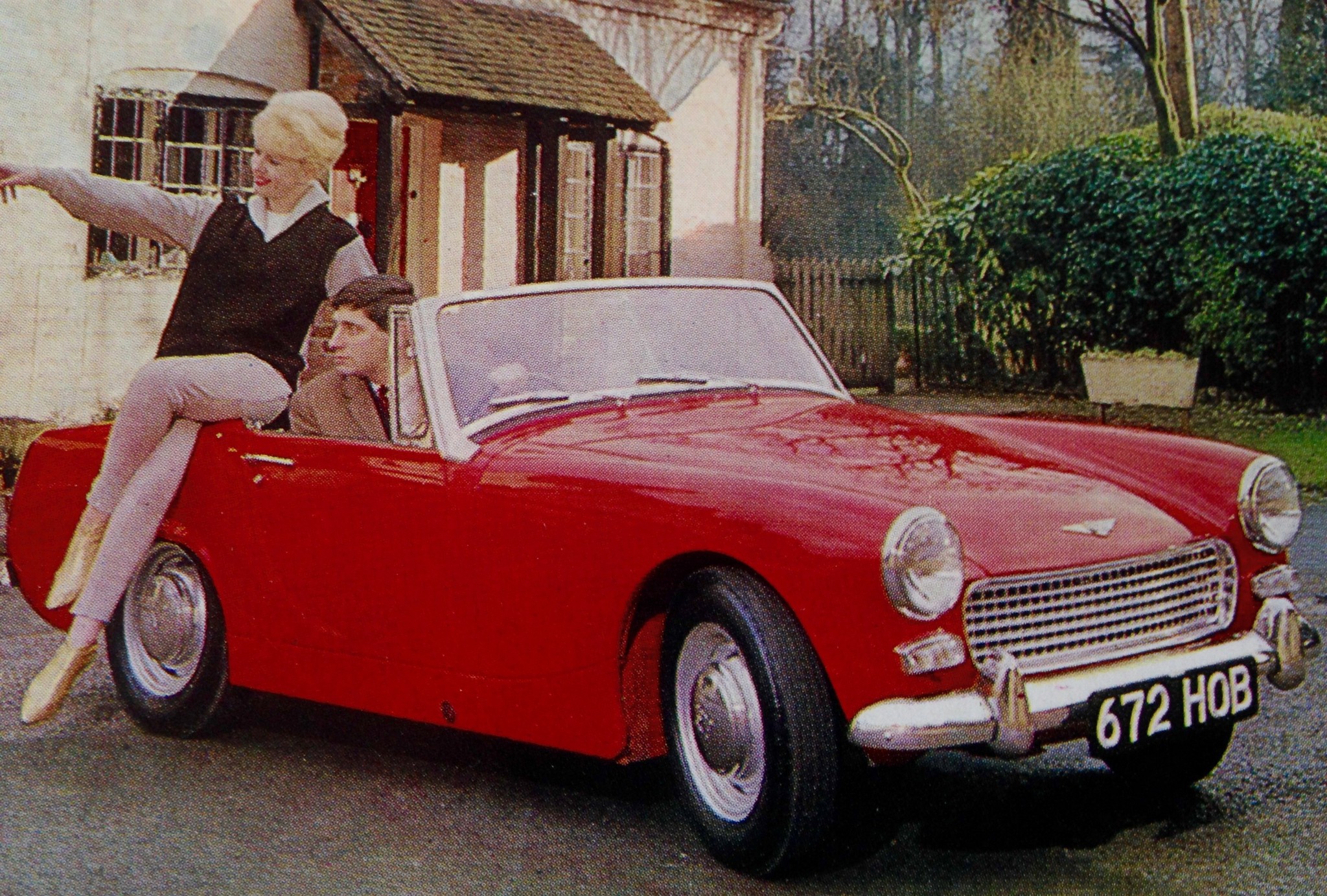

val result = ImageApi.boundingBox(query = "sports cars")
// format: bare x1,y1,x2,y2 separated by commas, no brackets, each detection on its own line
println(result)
2,271,1325,882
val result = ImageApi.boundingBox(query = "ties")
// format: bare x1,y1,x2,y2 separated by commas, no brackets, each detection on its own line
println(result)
374,384,391,442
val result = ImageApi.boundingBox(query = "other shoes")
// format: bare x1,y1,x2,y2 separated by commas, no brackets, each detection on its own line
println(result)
21,638,99,725
44,505,111,608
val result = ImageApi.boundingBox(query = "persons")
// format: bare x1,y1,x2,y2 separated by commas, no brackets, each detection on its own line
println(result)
285,275,535,452
1,90,384,728
